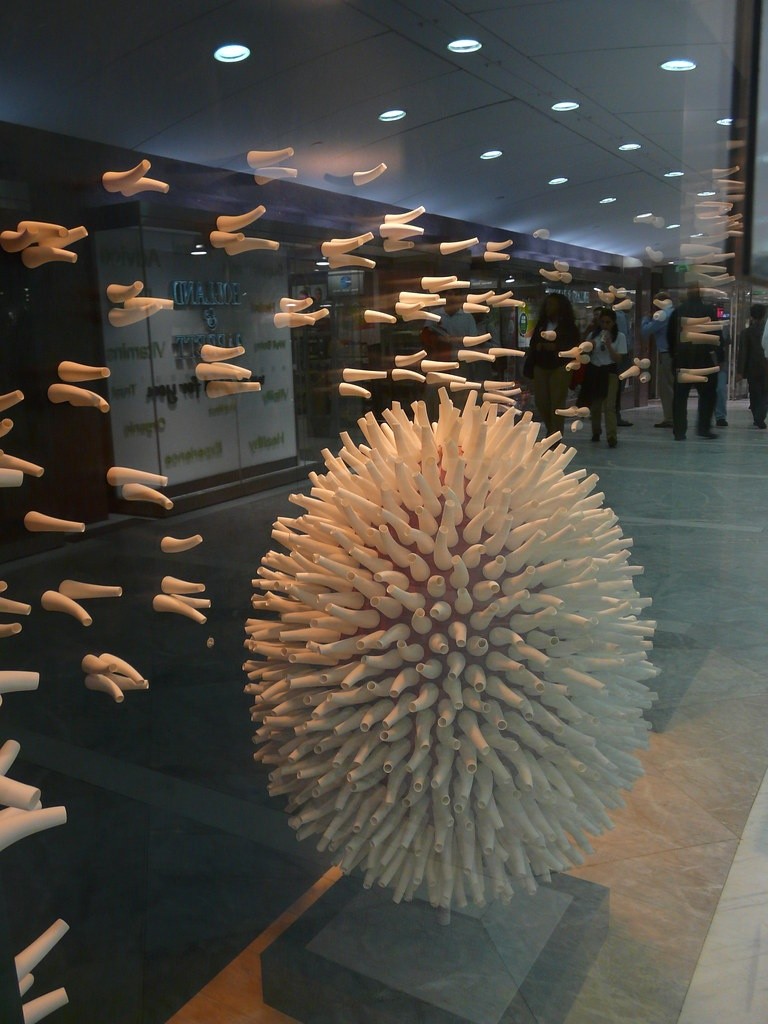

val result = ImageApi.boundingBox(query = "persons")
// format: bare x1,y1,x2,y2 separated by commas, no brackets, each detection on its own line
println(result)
525,292,579,447
426,288,478,416
585,282,768,447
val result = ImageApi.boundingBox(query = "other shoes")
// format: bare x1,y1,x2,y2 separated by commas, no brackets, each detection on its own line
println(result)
675,434,686,441
654,421,673,428
609,436,617,448
616,420,633,426
716,418,728,426
697,430,720,438
592,434,601,441
753,421,766,429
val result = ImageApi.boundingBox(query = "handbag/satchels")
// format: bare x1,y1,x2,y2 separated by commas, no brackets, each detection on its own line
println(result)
523,357,534,379
